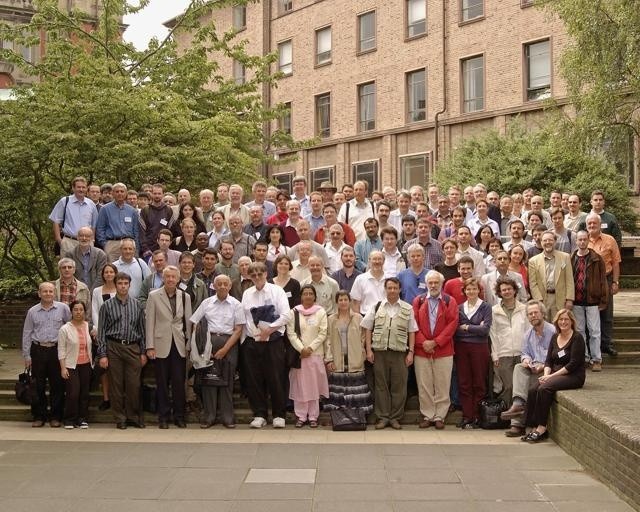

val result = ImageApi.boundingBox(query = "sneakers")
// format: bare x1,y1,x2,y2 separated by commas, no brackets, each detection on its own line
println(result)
374,416,479,430
500,405,525,420
32,417,318,430
585,347,618,372
98,400,111,412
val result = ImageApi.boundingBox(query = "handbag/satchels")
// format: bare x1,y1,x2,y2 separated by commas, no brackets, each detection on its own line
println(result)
54,197,70,256
195,358,232,387
15,365,39,405
289,310,303,368
479,399,511,429
330,406,368,431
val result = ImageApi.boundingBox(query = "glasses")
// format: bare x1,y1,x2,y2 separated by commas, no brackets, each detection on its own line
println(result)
61,266,74,270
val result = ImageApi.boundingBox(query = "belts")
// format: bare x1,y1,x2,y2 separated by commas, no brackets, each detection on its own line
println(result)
107,336,137,345
33,340,57,348
547,290,555,294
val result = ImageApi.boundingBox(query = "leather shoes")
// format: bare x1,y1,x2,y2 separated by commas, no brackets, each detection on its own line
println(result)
505,425,549,441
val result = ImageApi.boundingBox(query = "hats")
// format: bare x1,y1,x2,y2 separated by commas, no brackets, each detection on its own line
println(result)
317,181,338,194
